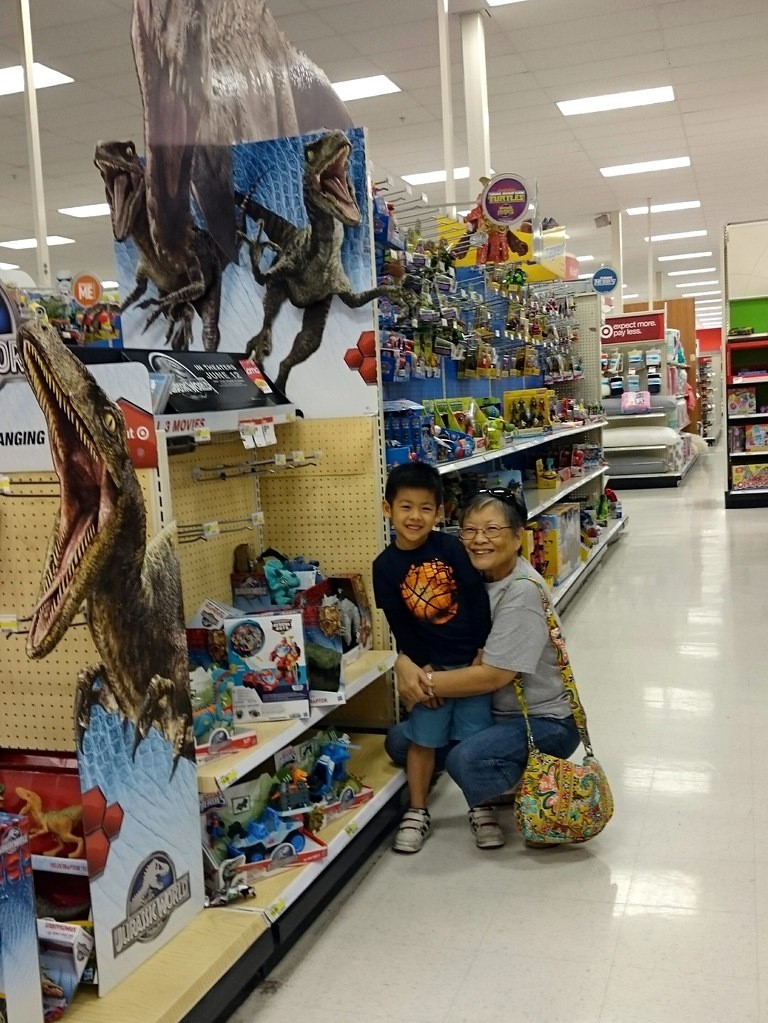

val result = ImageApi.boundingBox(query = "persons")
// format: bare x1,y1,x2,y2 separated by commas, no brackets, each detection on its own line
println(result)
371,462,506,855
382,489,581,811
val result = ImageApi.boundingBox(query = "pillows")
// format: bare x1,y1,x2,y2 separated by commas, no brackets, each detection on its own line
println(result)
600,390,680,474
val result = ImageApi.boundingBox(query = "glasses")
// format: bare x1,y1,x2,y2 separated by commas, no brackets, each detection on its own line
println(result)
457,523,513,540
466,487,523,520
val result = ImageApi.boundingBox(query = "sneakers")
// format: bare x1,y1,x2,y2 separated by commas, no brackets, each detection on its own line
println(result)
467,805,505,848
394,807,431,852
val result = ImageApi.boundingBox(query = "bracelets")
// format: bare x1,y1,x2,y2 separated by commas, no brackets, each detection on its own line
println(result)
426,671,434,696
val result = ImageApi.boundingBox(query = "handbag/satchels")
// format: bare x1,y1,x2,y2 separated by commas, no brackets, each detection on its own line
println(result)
513,749,613,844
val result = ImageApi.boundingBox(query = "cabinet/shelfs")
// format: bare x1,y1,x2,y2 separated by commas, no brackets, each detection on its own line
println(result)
698,355,716,428
603,412,666,450
433,413,628,611
190,646,402,946
728,339,768,495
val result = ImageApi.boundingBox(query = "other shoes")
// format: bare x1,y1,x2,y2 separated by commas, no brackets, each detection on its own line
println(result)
489,793,515,804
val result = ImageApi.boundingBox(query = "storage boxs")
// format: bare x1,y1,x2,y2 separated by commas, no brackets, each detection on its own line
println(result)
518,521,546,577
174,563,373,770
538,502,582,587
727,387,768,490
0,757,105,1023
